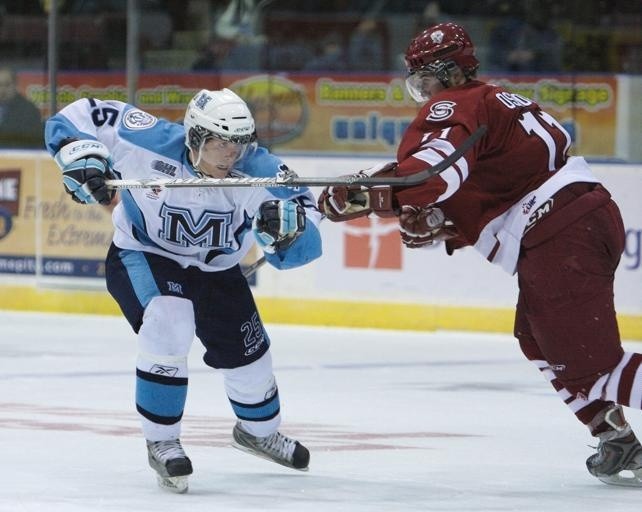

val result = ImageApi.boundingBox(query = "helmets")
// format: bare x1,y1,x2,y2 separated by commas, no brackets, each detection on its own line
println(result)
185,88,261,154
404,23,482,86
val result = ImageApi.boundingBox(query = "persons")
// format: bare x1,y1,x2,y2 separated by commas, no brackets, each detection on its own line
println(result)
317,20,642,478
0,65,45,151
42,89,327,477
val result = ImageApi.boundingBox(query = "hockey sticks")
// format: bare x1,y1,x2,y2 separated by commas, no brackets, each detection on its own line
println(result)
103,124,488,189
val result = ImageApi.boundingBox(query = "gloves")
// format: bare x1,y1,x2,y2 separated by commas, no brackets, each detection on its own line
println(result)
396,203,451,251
317,160,400,223
255,199,306,248
60,158,121,208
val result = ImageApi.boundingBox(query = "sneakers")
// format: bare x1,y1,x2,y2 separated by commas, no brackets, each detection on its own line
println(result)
232,421,311,469
146,436,193,478
584,428,640,479
